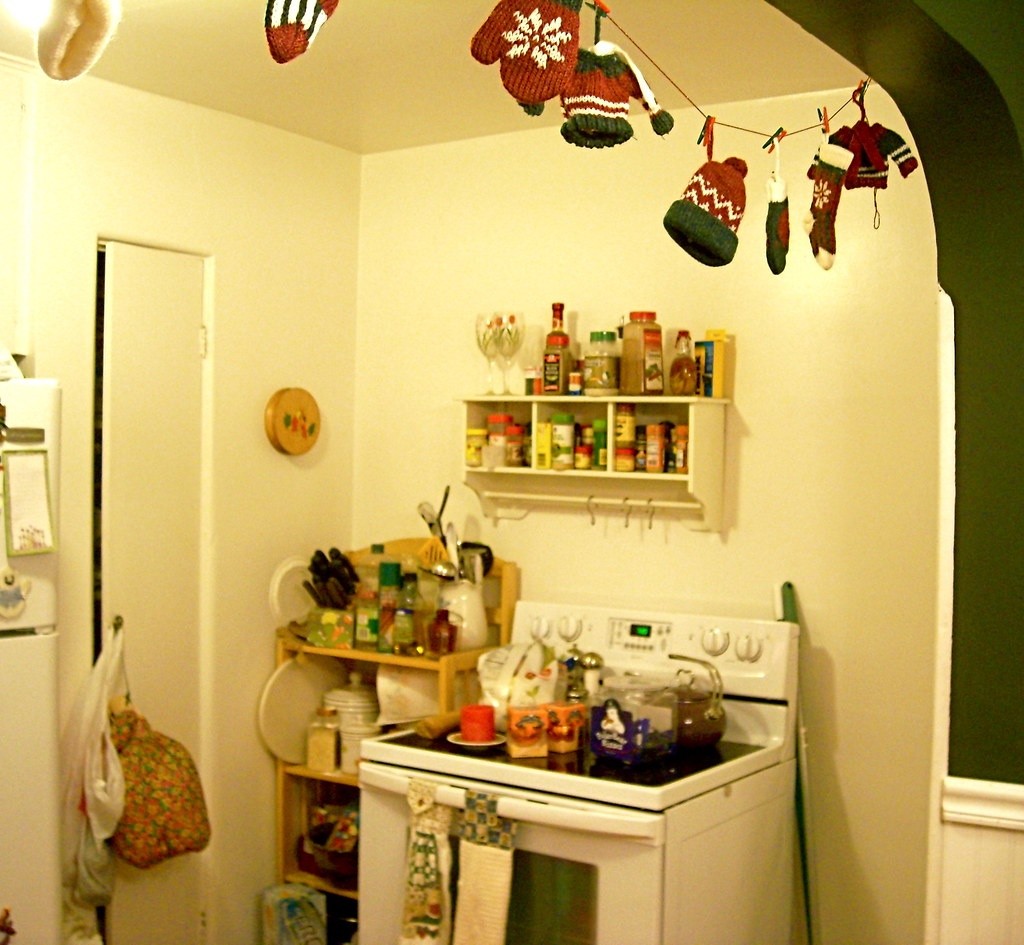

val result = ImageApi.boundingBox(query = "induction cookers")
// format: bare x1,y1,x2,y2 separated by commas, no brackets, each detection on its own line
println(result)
359,599,799,810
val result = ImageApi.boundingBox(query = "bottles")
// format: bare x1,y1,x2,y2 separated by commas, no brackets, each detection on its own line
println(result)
677,426,687,472
438,580,487,647
355,544,386,592
670,329,696,397
551,302,564,332
555,646,603,703
377,559,425,655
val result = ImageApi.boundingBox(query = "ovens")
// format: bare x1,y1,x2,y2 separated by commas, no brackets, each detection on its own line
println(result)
358,760,802,945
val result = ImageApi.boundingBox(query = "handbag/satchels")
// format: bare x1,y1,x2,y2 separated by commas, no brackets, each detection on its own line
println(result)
106,624,212,868
59,624,125,906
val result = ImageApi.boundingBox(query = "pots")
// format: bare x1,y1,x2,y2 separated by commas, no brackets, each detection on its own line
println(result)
592,668,680,755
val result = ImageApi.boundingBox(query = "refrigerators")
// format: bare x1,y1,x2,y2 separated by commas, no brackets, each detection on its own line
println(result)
0,380,76,945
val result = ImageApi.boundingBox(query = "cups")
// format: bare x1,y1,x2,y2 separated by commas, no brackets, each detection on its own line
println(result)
421,611,463,657
543,700,586,751
461,706,495,743
508,708,550,758
323,671,382,773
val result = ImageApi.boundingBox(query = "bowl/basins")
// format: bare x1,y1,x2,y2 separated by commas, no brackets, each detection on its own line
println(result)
307,822,360,891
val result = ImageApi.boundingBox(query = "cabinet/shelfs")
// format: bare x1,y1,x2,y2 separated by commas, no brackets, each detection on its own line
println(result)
453,394,731,531
274,538,523,899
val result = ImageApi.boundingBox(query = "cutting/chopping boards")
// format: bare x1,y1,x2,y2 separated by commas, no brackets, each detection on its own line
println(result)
259,654,348,764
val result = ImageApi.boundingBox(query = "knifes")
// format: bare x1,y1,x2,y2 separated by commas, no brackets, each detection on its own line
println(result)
303,546,360,611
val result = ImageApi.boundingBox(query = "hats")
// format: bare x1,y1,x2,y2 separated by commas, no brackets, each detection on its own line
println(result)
664,156,748,267
559,49,675,149
38,0,124,82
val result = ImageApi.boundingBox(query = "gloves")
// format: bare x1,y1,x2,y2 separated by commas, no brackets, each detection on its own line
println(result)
469,0,585,102
767,176,790,275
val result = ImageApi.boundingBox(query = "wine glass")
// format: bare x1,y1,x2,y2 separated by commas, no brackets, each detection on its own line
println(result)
475,313,520,396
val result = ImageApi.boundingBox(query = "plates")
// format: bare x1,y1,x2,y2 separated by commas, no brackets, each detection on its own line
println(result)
449,733,504,746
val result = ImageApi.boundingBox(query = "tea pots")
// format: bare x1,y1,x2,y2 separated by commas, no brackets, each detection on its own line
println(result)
666,653,727,747
